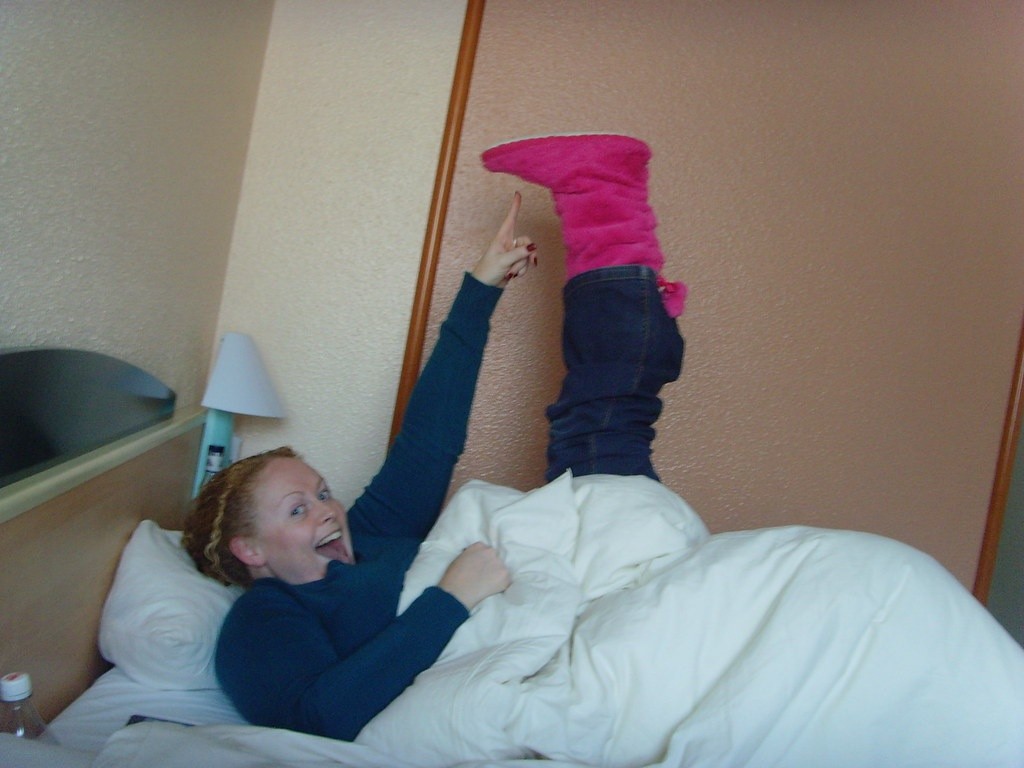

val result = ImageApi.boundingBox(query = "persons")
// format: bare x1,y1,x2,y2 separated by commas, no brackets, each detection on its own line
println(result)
178,133,687,744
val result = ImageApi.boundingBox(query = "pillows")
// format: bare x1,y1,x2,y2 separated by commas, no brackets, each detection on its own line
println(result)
95,519,246,691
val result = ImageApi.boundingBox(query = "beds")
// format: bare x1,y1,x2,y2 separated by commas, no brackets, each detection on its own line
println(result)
1,348,1023,768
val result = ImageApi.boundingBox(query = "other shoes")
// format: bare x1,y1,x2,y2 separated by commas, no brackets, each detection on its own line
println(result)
484,132,688,320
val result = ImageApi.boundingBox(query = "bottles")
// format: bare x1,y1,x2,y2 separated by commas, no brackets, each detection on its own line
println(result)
200,444,225,486
0,670,59,750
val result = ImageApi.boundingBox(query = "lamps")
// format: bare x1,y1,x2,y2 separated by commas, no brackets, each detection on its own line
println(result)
192,332,285,498
